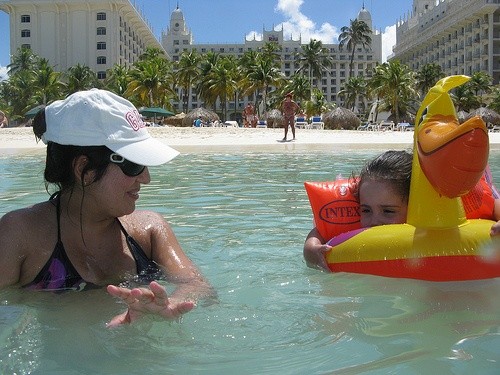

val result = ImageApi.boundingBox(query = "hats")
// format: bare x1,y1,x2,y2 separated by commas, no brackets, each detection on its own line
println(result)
40,87,181,168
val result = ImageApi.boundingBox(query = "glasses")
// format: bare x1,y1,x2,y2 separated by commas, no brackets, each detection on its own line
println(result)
109,153,146,177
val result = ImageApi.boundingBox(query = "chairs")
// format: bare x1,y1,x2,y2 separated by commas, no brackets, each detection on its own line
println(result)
358,120,380,130
255,120,269,128
397,123,410,131
308,116,325,130
294,117,309,131
210,120,223,128
192,119,207,127
379,120,396,131
224,120,240,128
486,123,500,133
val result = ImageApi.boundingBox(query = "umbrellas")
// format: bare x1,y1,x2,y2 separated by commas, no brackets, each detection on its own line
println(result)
140,106,174,127
456,107,500,127
322,106,361,129
261,108,285,128
165,107,219,127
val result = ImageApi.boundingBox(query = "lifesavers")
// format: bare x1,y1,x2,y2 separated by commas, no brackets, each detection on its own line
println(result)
322,74,500,282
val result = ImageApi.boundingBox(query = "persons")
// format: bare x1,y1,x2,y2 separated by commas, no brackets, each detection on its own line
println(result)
297,109,306,118
0,88,219,331
194,117,221,128
242,102,258,128
304,150,500,272
281,93,300,141
